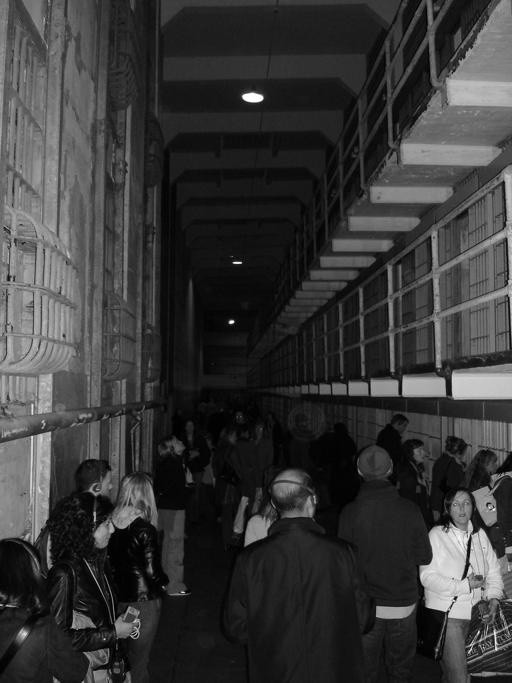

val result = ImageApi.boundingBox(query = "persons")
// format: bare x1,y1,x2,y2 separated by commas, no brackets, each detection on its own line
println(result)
376,413,410,487
463,449,498,496
215,426,243,523
151,436,192,595
70,458,113,499
399,439,429,503
95,472,188,683
340,445,433,683
237,427,253,483
490,450,512,561
431,436,467,513
419,487,504,683
232,410,245,424
0,537,90,683
221,469,377,683
43,491,141,683
253,422,274,490
177,420,211,520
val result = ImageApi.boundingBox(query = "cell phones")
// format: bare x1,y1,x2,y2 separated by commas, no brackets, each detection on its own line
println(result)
123,606,139,621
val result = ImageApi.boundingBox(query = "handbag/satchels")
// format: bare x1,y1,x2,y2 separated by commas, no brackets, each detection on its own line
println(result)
466,597,512,680
414,535,473,662
472,477,508,527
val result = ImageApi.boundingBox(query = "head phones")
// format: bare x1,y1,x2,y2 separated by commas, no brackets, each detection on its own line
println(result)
0,538,42,578
268,480,319,506
92,457,105,492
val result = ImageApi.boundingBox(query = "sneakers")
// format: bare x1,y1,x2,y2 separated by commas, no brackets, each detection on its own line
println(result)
168,587,192,596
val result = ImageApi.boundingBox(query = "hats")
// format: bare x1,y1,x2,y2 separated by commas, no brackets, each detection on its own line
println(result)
356,445,391,478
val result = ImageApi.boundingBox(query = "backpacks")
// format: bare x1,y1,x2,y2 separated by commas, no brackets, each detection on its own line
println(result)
30,518,53,578
